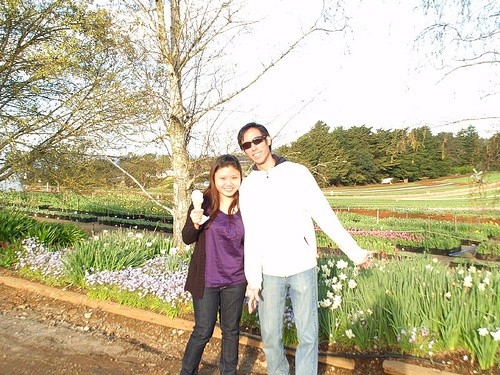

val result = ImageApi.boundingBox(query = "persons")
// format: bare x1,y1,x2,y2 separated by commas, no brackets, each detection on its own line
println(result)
237,122,373,375
180,154,248,375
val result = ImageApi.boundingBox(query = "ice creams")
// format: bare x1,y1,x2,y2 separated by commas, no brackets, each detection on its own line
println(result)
191,189,204,208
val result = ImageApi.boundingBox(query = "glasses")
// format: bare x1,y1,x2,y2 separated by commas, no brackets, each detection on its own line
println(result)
240,135,266,151
